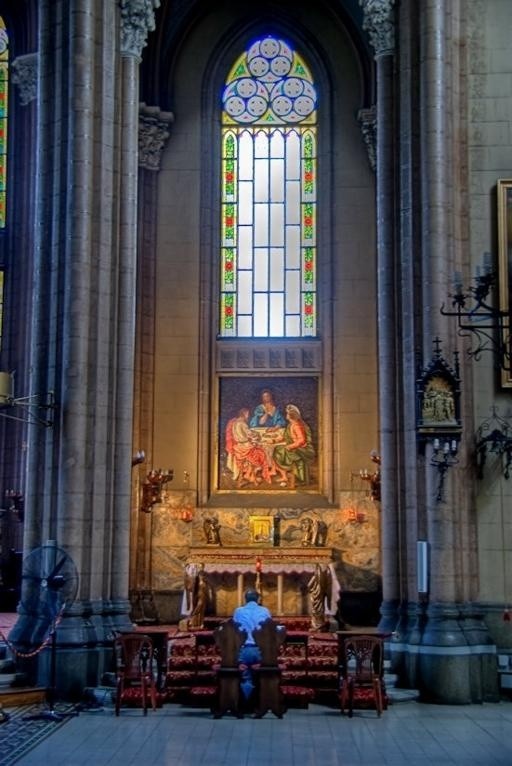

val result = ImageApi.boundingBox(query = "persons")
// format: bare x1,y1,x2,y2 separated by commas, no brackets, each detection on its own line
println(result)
187,563,213,631
307,563,332,632
233,589,271,700
225,389,315,487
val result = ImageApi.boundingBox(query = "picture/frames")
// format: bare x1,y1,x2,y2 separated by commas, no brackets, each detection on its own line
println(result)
495,177,512,393
247,514,276,549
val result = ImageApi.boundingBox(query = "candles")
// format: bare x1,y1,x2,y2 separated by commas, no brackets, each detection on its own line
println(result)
444,442,450,453
434,439,441,449
451,440,456,450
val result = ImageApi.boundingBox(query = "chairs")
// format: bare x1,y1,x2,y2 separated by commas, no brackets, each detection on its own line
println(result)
111,633,160,718
338,635,389,721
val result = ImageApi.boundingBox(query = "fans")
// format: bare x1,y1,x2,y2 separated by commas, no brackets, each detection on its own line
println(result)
18,547,84,722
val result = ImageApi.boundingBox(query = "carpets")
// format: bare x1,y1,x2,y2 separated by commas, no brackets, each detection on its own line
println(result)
1,696,81,766
